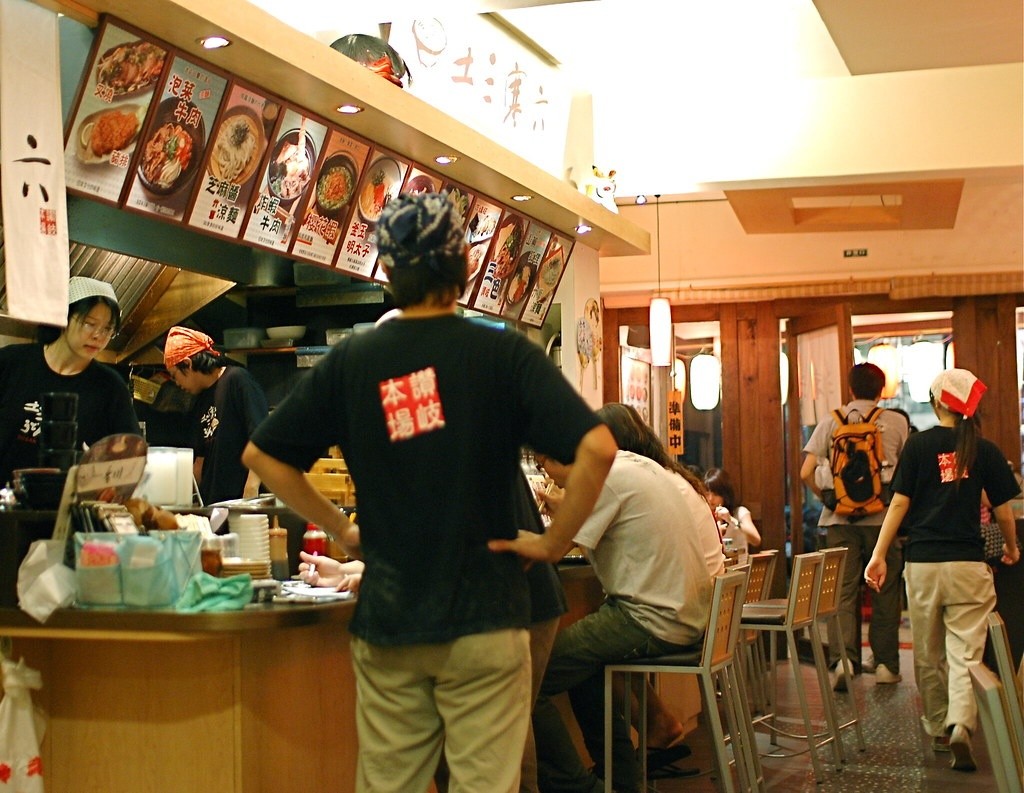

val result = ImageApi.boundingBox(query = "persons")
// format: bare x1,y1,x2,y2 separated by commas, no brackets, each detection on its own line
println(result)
800,363,909,692
863,369,1021,772
241,192,618,793
164,324,270,535
883,406,992,617
0,274,144,609
297,458,570,793
525,401,761,793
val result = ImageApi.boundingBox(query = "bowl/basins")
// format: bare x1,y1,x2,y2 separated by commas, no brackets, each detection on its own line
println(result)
267,325,306,339
206,105,265,189
136,97,206,196
316,150,359,213
358,157,401,223
269,128,317,207
13,468,68,510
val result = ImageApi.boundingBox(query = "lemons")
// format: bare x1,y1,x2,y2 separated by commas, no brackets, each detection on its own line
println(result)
81,122,95,148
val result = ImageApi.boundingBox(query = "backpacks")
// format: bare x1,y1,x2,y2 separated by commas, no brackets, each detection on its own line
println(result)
826,406,886,522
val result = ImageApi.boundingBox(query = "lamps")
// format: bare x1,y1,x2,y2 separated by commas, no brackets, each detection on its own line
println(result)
649,193,673,367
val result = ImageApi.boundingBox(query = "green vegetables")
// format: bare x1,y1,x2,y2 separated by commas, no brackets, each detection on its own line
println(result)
165,136,179,157
232,122,250,146
372,169,385,184
318,166,353,209
505,222,520,257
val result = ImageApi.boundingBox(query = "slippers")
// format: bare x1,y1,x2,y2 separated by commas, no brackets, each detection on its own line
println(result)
635,744,700,779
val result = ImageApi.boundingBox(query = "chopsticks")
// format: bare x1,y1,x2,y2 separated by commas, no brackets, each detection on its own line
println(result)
302,200,315,224
537,482,554,513
258,192,296,223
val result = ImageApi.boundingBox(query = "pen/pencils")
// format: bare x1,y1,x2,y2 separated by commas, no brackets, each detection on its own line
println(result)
308,550,318,576
139,521,146,533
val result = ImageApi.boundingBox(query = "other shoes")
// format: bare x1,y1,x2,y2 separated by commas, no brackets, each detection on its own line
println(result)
831,658,854,693
930,736,950,752
875,663,902,683
948,727,977,772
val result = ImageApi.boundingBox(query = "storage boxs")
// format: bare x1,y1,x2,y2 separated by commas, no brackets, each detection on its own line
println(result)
223,328,265,349
326,328,353,346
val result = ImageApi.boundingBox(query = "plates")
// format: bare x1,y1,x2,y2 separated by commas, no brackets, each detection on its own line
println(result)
467,244,488,282
222,558,273,581
506,251,538,306
491,214,524,278
97,40,158,99
404,176,435,196
76,103,145,165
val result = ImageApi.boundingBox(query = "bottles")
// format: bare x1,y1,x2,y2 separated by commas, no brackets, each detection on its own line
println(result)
269,516,291,582
303,521,327,557
723,538,733,551
200,536,221,578
327,535,349,564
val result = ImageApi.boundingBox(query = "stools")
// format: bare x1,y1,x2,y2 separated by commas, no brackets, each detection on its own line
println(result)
605,544,868,793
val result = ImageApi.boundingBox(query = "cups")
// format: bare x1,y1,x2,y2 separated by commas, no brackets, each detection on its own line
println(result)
39,448,76,470
262,99,277,125
41,421,77,449
40,392,78,421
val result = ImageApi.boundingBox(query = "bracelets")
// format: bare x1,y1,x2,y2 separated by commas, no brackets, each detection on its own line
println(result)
735,520,742,530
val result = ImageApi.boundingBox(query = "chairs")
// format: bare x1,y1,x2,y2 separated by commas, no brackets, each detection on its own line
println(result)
985,611,1024,761
969,662,1024,793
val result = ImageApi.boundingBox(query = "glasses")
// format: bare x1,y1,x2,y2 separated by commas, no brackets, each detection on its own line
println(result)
536,455,548,473
73,315,117,340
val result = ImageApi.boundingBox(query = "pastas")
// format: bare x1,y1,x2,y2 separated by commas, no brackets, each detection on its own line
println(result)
281,154,311,198
161,159,184,186
212,117,256,181
